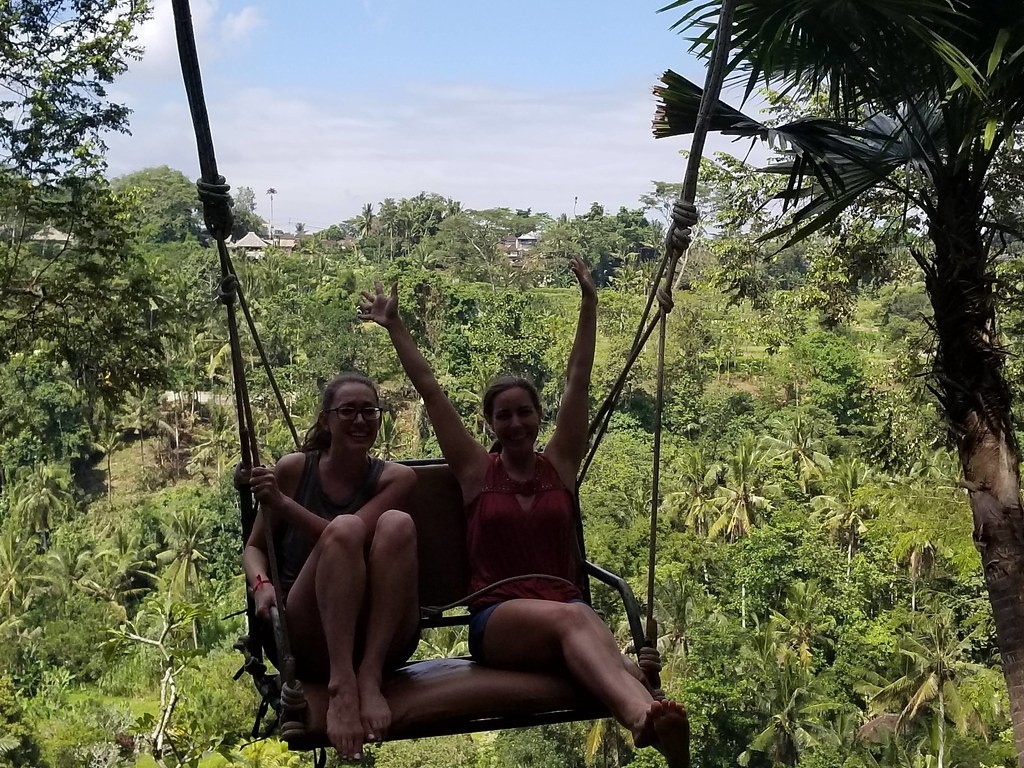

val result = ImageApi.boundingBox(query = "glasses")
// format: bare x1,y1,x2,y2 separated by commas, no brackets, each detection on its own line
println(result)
325,406,384,421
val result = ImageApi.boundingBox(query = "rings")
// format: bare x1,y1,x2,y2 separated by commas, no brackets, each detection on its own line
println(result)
256,485,259,490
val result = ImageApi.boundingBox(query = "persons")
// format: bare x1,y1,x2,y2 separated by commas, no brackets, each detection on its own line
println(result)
356,253,692,768
240,377,420,762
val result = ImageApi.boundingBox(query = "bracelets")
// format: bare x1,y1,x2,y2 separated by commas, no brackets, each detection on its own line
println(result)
253,575,271,593
